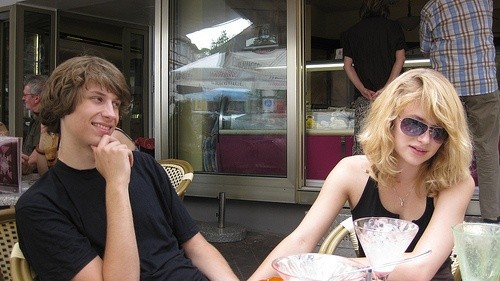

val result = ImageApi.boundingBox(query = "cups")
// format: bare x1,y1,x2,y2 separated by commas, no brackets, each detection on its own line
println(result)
271,253,368,281
451,223,500,281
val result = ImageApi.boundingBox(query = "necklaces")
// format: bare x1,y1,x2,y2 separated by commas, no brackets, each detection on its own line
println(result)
396,183,415,207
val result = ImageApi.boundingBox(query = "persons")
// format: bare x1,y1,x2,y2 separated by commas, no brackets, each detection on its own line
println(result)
15,56,239,281
343,0,408,156
21,75,61,173
246,68,476,281
419,0,500,223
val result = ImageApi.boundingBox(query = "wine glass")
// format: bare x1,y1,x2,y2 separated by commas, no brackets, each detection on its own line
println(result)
353,217,419,281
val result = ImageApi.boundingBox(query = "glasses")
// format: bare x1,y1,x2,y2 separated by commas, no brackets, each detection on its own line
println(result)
21,92,35,96
397,115,450,145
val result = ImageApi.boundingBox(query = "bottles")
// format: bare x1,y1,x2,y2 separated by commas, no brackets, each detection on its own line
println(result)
261,90,285,113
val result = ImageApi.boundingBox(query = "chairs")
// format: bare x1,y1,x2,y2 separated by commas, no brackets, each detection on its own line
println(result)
156,158,192,198
0,208,37,281
